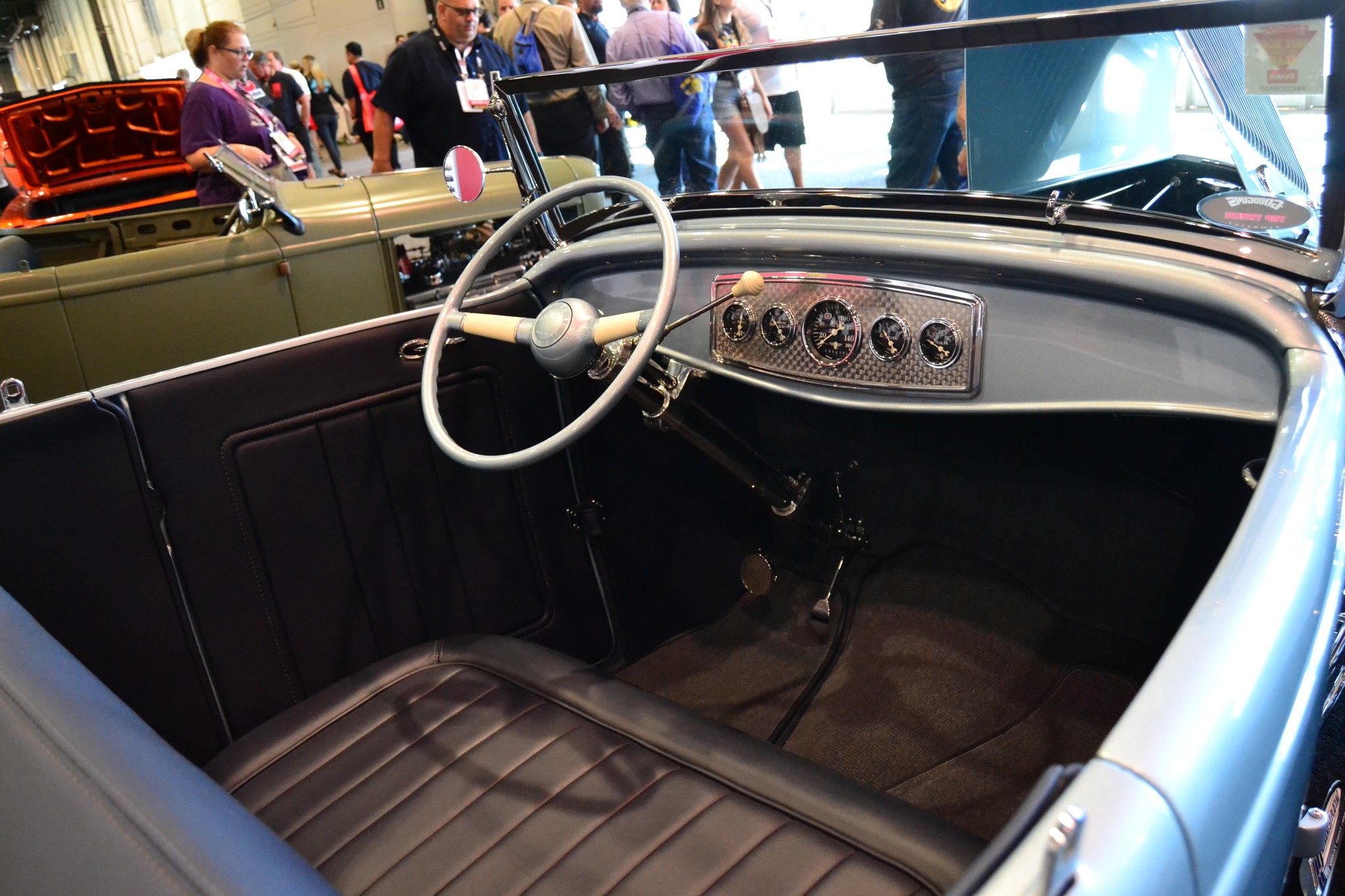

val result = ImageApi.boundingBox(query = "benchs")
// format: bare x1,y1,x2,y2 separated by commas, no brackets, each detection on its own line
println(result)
0,589,988,896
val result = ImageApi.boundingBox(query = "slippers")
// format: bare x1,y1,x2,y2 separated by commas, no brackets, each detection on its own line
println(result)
328,168,346,177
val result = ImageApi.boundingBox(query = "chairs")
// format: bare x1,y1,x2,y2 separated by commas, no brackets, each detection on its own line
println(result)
0,233,46,272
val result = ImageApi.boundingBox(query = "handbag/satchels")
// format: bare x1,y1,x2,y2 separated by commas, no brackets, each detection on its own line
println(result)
740,91,769,136
361,90,405,132
666,11,709,127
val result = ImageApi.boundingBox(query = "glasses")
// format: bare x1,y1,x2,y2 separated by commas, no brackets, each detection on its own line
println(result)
215,46,254,61
443,1,484,17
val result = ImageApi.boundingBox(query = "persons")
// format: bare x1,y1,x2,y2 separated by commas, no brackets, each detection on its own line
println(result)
573,0,636,209
861,0,967,199
687,0,774,193
247,50,315,183
497,0,521,19
921,74,970,190
732,0,808,193
264,52,327,179
329,92,357,145
371,0,546,169
290,60,305,75
343,41,404,171
301,55,352,178
605,0,722,199
648,0,681,14
388,36,413,145
185,28,274,114
179,21,298,207
476,8,493,41
492,0,609,230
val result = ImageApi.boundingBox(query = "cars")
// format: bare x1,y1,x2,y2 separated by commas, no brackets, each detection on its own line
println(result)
0,0,1345,894
0,127,608,409
0,76,244,229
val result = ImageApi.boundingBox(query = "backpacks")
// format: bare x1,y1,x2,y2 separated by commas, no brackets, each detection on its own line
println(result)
511,3,554,98
